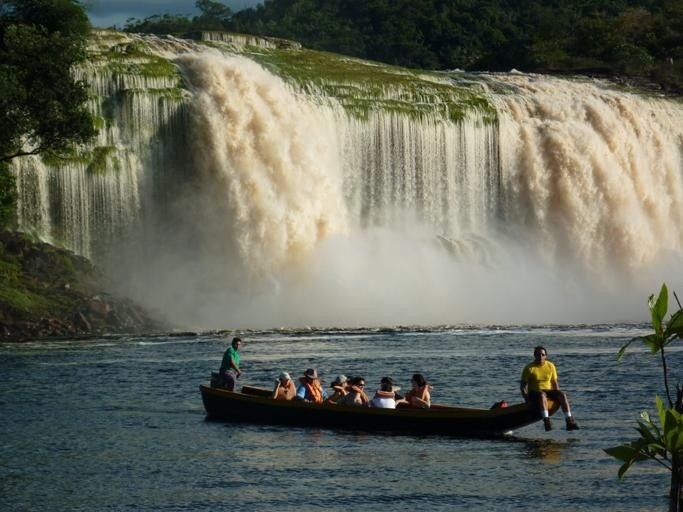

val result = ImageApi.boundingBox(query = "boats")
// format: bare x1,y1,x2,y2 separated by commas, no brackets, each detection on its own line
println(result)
199,383,560,434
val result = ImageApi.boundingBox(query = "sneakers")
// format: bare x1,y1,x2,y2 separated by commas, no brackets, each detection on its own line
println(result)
543,417,553,432
566,416,579,431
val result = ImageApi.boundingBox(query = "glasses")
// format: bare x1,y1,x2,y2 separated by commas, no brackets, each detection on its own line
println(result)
358,384,365,386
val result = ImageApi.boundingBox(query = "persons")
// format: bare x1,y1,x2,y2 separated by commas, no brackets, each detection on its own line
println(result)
520,346,580,432
220,337,242,392
271,368,431,409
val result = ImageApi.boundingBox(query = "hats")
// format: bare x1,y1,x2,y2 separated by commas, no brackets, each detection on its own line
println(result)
278,371,291,380
336,374,347,384
304,369,318,379
